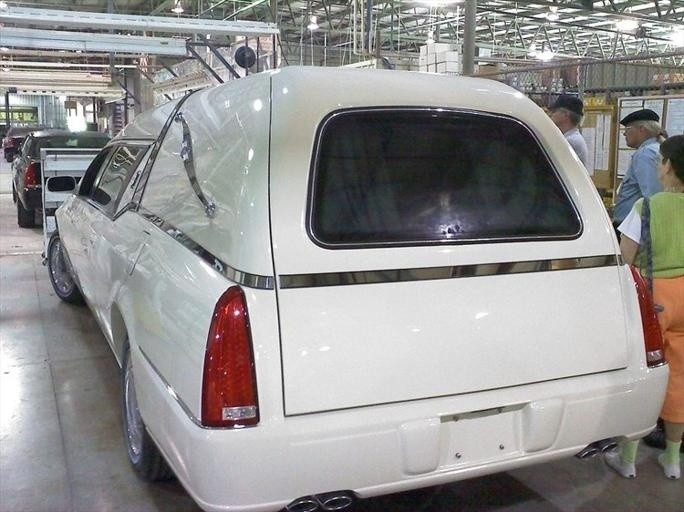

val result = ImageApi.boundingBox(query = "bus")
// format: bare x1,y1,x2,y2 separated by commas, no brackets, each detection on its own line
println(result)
0,104,38,147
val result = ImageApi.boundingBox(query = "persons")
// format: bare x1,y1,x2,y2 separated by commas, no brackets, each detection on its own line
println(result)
602,135,684,481
612,107,666,243
547,94,588,170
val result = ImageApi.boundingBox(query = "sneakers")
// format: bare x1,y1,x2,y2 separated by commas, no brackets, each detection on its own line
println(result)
657,453,681,480
604,451,636,479
643,418,683,455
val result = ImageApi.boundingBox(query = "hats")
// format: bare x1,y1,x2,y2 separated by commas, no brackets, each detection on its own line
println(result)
547,95,583,116
619,109,659,126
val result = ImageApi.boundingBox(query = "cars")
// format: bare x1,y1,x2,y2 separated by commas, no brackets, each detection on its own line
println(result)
14,130,112,224
50,66,671,512
4,123,44,162
